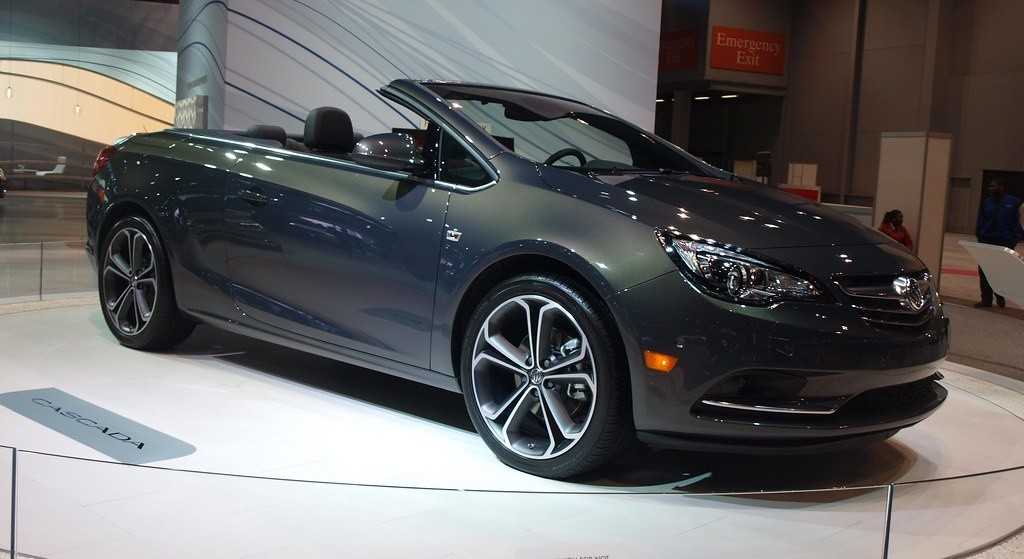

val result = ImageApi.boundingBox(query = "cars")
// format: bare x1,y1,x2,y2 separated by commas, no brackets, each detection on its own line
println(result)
0,168,8,198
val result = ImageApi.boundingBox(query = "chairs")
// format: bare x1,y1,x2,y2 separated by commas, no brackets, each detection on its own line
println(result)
36,155,73,176
304,106,355,159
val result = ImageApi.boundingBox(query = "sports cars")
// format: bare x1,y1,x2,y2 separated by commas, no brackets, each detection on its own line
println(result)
83,78,951,480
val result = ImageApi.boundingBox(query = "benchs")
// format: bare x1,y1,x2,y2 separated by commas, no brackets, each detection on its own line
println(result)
245,124,363,153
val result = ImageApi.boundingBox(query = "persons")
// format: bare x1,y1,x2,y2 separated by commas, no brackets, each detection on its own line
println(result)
878,210,913,251
975,180,1024,308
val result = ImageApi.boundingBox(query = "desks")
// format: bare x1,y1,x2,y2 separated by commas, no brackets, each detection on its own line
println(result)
13,168,37,189
